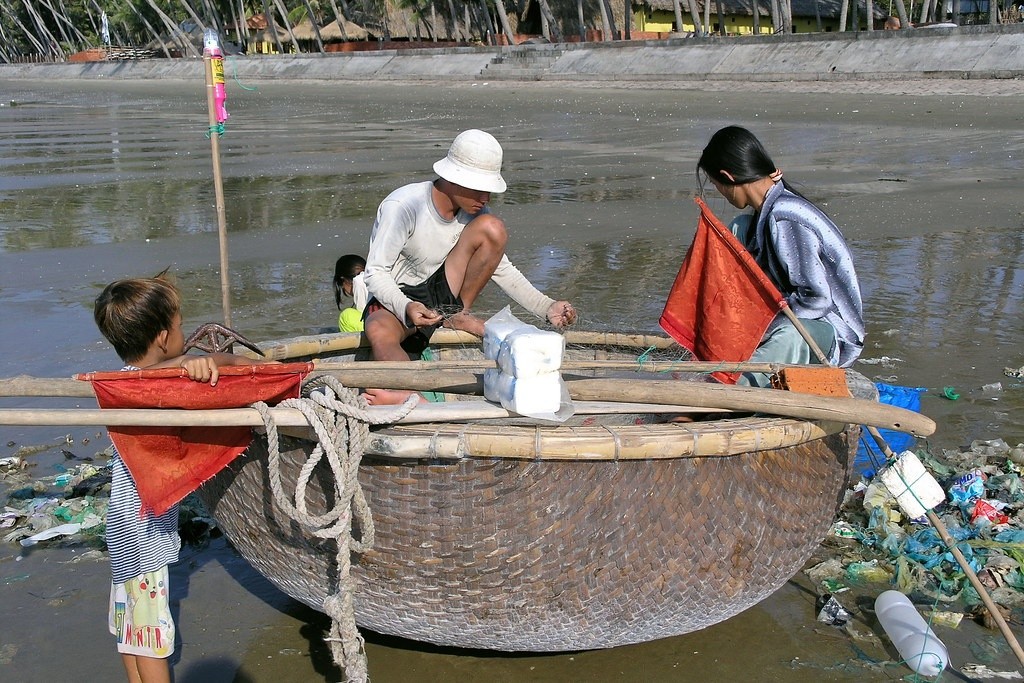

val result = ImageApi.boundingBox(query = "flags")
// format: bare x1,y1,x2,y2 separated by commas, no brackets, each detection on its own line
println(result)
90,361,315,518
658,198,788,386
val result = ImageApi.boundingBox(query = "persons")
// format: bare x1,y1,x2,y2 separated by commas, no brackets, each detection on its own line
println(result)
695,126,865,425
361,129,577,405
333,255,374,331
94,279,219,683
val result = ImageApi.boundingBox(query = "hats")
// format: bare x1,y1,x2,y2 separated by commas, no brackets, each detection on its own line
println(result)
433,129,507,193
